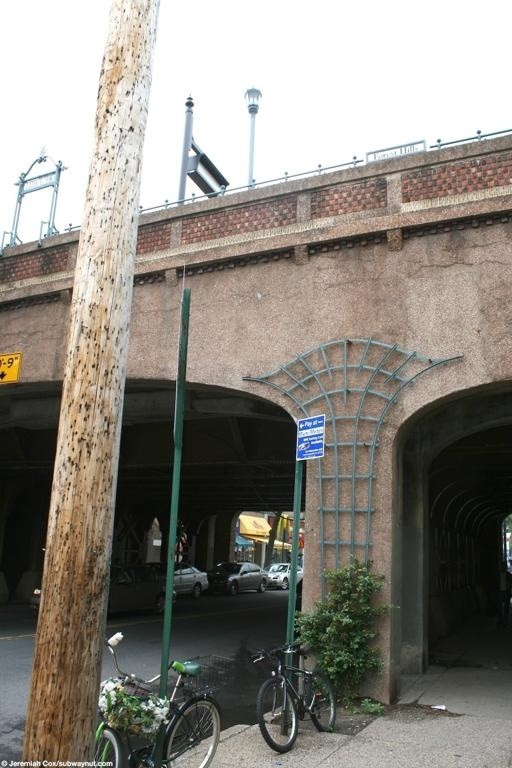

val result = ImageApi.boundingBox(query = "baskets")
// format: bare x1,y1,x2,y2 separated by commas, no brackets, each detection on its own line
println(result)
183,651,235,696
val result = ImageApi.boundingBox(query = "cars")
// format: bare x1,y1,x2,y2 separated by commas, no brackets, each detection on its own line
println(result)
208,561,268,596
263,562,303,590
31,562,208,614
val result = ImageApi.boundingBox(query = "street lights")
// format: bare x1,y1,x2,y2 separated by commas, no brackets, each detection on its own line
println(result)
244,87,263,190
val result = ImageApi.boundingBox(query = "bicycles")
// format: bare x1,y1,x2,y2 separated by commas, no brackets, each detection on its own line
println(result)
249,642,336,753
94,632,236,768
